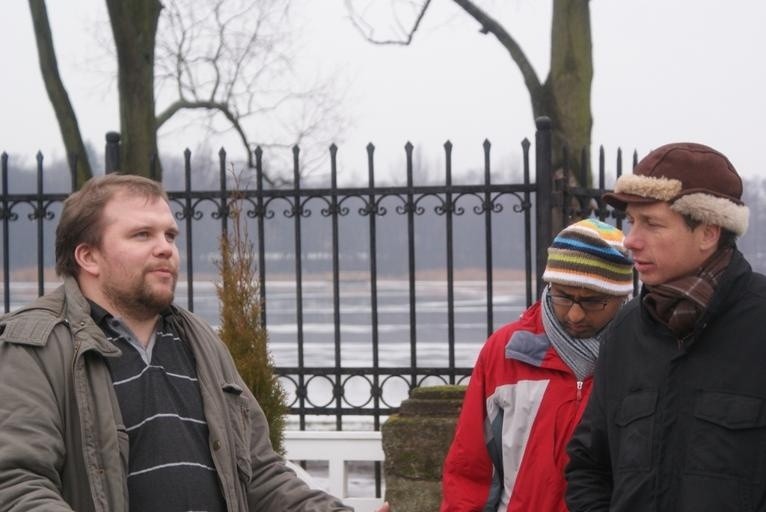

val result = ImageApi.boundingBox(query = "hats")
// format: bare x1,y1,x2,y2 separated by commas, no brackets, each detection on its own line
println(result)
601,142,749,238
541,219,635,297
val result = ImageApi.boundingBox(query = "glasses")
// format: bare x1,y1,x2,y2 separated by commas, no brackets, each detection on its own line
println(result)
545,287,612,311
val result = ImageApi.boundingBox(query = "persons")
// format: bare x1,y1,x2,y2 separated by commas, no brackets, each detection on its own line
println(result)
440,218,644,512
564,143,766,512
0,171,389,512
